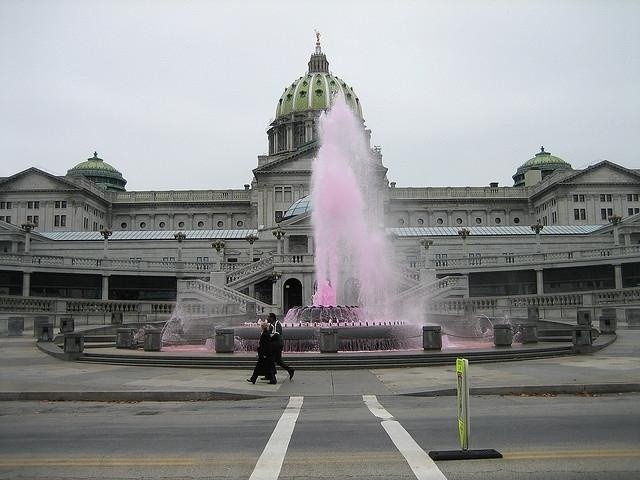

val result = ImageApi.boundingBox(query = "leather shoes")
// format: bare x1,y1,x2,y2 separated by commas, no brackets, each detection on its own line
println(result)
246,379,255,384
290,367,295,380
267,382,276,384
261,377,270,380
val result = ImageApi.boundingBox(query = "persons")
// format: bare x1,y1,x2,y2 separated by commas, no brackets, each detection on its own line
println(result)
261,313,294,381
246,322,277,384
